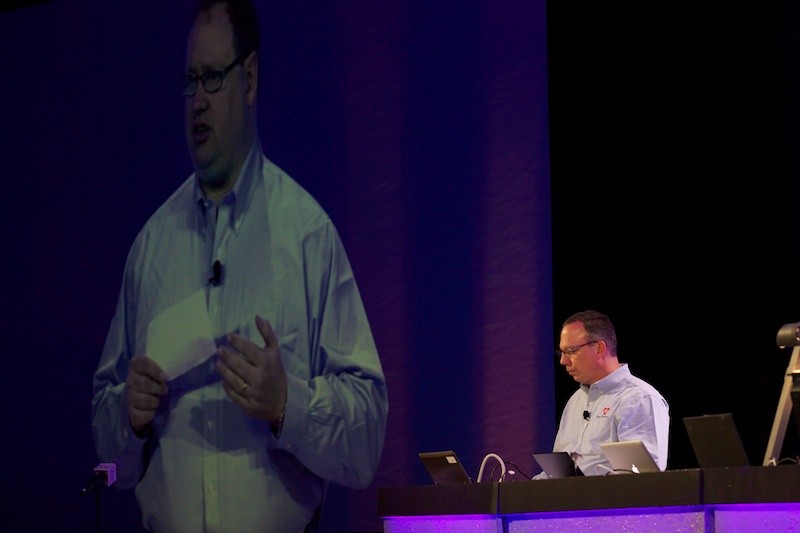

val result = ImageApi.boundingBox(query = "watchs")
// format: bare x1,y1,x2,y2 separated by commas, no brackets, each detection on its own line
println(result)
275,389,288,438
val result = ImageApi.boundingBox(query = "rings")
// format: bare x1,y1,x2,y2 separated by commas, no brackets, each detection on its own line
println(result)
238,384,248,398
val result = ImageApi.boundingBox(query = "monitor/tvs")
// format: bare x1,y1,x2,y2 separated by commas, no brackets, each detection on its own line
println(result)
598,440,660,474
534,452,586,478
418,450,473,484
683,413,749,471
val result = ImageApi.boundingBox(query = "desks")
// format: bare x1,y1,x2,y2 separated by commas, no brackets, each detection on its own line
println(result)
375,464,800,533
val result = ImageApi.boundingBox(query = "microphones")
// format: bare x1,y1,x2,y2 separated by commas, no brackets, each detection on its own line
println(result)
583,411,589,419
80,455,121,492
208,260,222,287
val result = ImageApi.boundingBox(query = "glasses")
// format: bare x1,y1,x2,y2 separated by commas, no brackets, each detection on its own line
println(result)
177,50,248,98
555,339,606,355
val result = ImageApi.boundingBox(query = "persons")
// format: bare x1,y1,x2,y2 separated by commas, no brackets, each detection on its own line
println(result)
532,310,671,482
94,0,387,533
